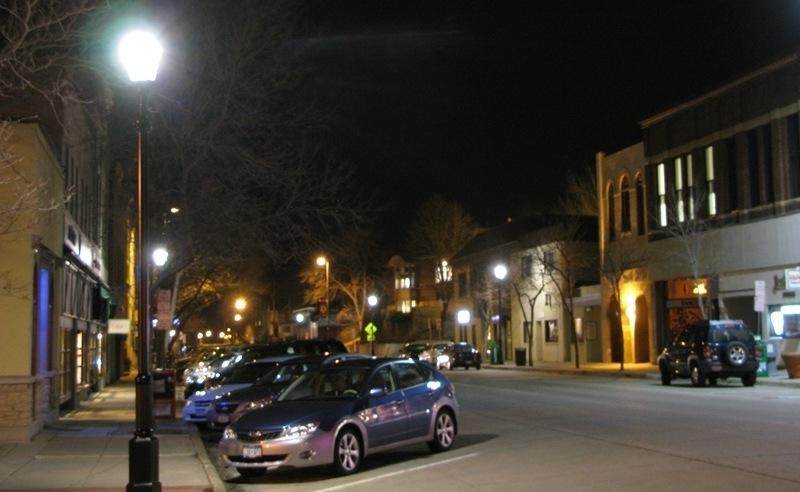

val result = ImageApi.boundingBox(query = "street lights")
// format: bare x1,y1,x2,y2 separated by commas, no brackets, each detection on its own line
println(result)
367,294,378,356
315,256,331,337
493,263,510,366
114,26,168,492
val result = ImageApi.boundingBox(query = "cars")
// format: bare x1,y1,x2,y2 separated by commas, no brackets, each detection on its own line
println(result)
214,352,464,478
177,335,351,401
396,341,430,363
180,352,323,433
417,343,457,370
436,340,486,372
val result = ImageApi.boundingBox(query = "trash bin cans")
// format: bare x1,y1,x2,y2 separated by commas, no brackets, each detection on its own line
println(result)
515,348,526,366
149,368,177,420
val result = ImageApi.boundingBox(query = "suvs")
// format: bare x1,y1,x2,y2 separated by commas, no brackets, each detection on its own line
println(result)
655,317,762,390
